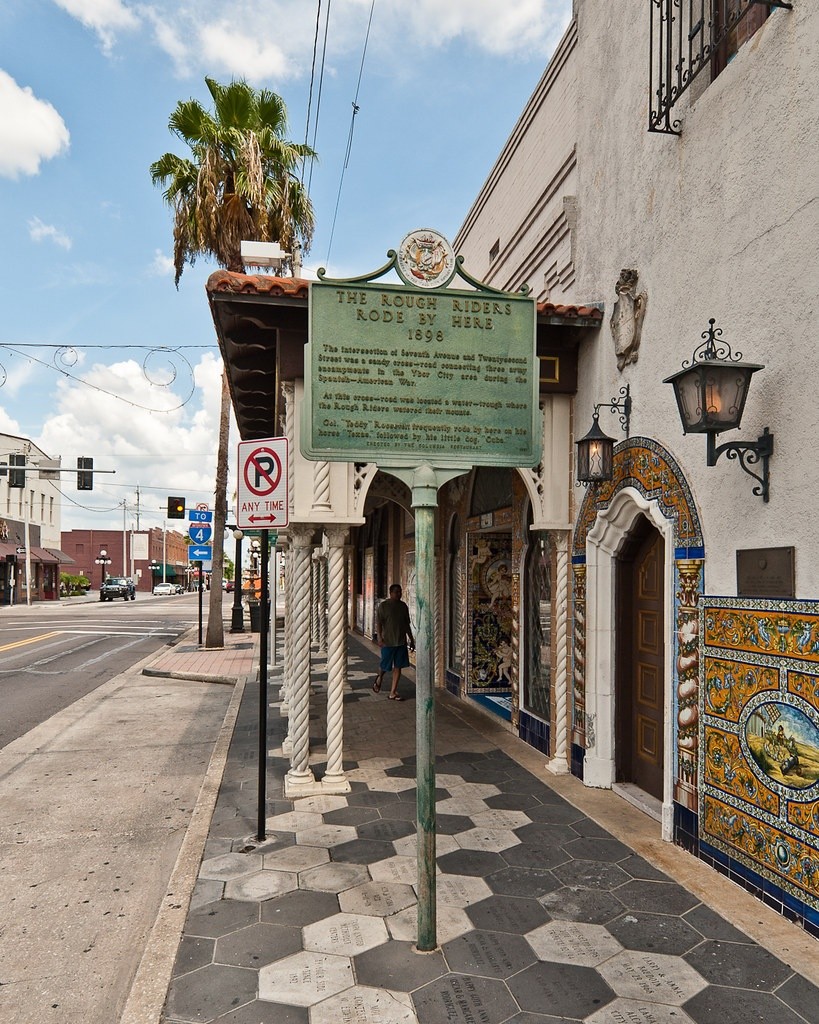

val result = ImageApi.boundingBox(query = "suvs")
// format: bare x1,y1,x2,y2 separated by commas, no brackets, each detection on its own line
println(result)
99,575,136,601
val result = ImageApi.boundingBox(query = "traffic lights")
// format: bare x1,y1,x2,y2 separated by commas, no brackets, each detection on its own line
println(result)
167,496,186,519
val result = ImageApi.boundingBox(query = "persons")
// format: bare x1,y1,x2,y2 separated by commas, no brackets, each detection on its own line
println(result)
372,584,416,701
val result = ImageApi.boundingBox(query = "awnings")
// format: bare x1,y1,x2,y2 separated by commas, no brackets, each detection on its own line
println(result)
154,563,177,576
0,543,75,564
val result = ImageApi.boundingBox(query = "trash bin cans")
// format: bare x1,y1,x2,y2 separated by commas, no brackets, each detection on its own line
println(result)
247,600,271,632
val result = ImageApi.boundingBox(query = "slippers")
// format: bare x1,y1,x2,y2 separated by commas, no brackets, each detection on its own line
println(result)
372,675,382,693
388,694,403,701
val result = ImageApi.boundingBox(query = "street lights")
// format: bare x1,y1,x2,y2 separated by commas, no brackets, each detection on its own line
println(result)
223,524,245,634
247,540,261,575
185,563,194,592
94,549,113,599
148,559,161,594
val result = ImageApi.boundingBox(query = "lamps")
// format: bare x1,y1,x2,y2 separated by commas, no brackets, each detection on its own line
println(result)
663,318,773,504
574,383,632,497
240,241,292,268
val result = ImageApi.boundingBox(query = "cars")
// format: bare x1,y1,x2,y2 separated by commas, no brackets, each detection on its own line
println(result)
173,584,185,594
193,580,207,592
153,582,176,596
222,579,235,593
